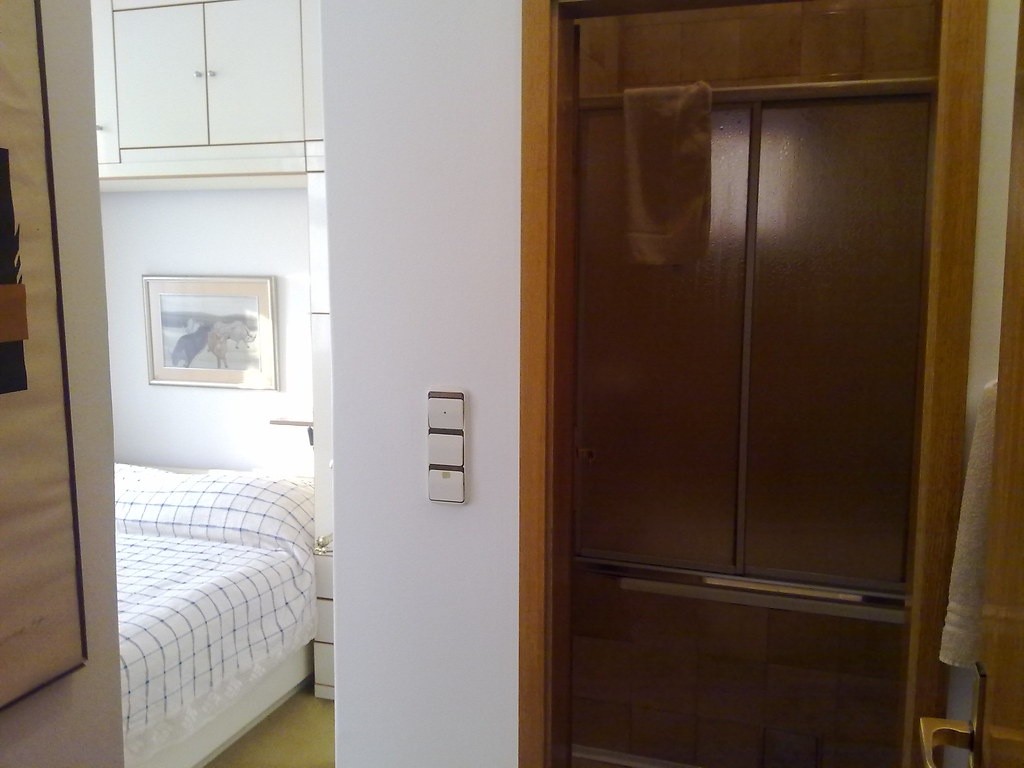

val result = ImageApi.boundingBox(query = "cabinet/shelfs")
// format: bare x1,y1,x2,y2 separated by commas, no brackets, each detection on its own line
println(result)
300,0,324,142
90,0,122,165
110,1,304,150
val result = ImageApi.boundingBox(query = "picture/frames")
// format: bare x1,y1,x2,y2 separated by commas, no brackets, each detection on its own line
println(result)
141,275,281,394
0,0,93,709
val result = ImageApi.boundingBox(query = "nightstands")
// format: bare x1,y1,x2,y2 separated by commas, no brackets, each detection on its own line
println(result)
311,549,334,700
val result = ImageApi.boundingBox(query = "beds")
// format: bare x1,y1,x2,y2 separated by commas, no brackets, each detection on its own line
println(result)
115,463,319,768
